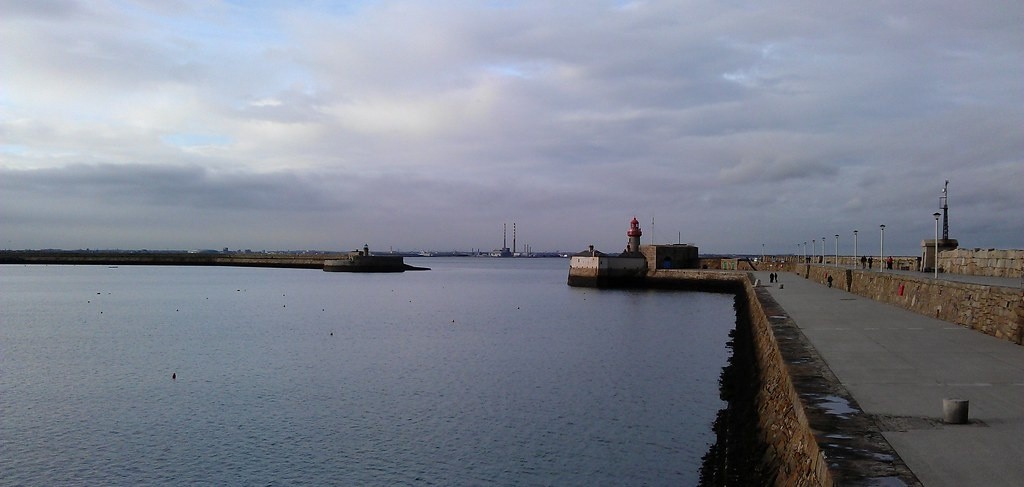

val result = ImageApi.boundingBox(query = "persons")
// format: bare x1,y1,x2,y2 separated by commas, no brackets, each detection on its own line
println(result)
770,273,778,283
828,275,833,288
861,255,867,269
868,256,873,269
886,256,893,270
806,256,811,263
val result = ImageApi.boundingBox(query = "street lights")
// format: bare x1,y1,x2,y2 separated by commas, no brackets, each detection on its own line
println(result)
822,237,826,265
853,230,858,269
798,244,800,263
813,240,815,264
933,212,942,279
762,243,765,263
879,224,886,273
835,234,839,268
804,242,807,264
